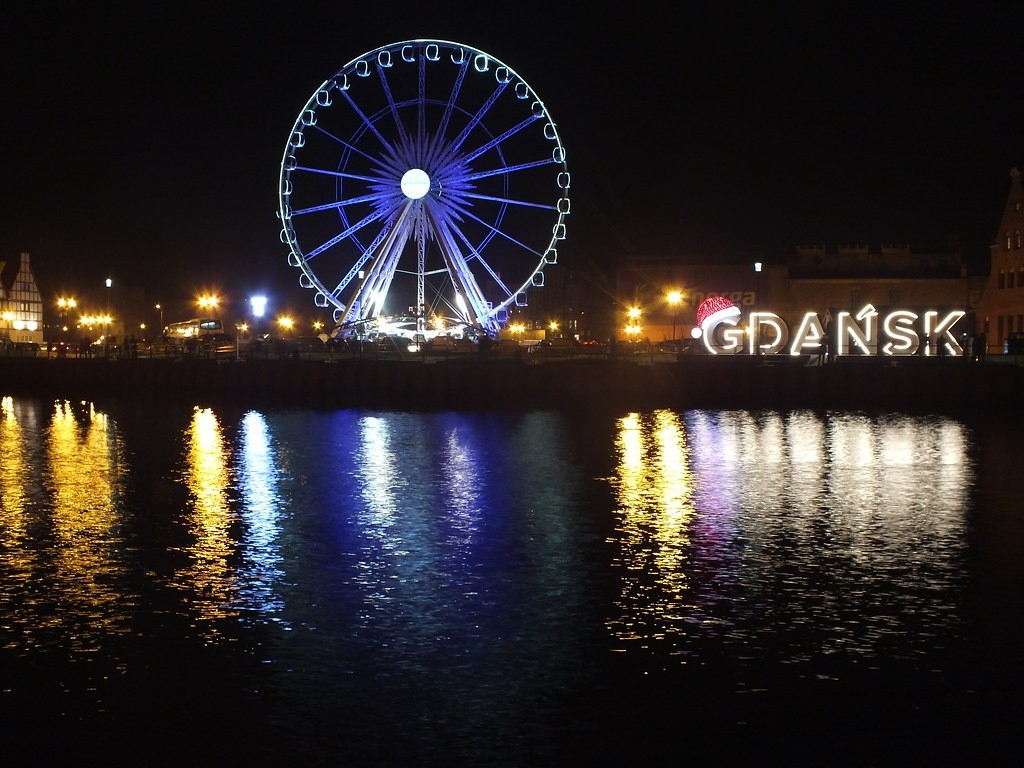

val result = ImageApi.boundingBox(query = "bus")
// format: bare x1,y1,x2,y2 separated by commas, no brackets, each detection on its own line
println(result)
168,319,224,340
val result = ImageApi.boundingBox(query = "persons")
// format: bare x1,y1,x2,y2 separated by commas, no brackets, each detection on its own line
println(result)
78,334,92,359
123,335,136,350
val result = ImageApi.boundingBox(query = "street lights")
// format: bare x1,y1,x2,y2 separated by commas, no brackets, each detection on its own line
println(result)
755,261,762,353
157,304,163,334
200,297,216,340
235,325,248,359
669,292,681,344
105,278,112,356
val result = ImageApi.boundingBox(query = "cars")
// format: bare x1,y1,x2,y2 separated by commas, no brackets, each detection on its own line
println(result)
251,328,474,354
